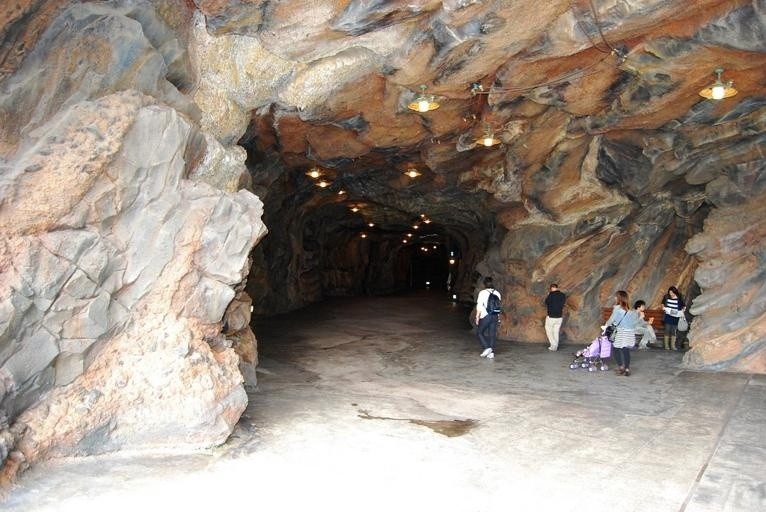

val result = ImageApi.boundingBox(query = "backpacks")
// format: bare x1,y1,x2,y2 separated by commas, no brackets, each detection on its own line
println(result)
483,288,502,314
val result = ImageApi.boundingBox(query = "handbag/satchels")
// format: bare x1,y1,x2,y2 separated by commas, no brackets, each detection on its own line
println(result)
605,324,617,343
497,313,501,325
678,317,689,331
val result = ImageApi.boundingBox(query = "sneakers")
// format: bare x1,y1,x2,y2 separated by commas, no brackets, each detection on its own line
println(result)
487,352,495,359
547,345,560,352
479,347,493,357
624,367,631,376
614,367,626,375
637,340,659,352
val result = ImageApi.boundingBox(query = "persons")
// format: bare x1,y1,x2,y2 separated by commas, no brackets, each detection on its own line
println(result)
600,290,636,375
475,276,502,359
633,299,657,349
660,286,687,350
545,284,566,351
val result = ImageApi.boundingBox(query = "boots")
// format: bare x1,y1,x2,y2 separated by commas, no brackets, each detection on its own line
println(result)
670,335,678,351
663,335,671,351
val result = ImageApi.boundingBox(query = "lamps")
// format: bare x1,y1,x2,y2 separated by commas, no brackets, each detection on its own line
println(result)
471,123,504,148
302,164,327,180
400,166,426,178
404,81,442,115
697,66,739,102
313,181,332,188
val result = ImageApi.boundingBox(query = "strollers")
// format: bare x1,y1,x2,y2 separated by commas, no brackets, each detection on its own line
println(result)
567,325,616,373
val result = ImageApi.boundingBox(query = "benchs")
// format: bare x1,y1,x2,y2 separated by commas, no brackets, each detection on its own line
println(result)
599,305,667,342
